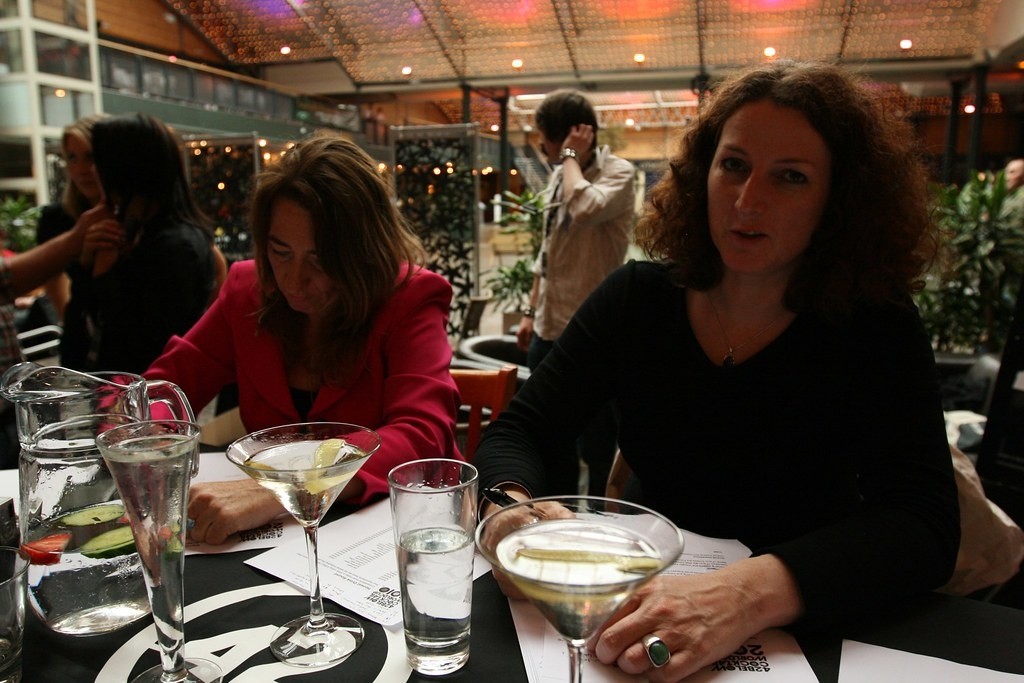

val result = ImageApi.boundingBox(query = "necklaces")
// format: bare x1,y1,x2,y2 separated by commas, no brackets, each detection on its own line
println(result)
702,290,789,367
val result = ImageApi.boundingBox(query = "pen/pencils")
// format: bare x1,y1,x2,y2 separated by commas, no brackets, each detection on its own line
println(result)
478,485,549,521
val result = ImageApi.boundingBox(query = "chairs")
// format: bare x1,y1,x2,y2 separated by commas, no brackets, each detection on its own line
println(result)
448,365,519,469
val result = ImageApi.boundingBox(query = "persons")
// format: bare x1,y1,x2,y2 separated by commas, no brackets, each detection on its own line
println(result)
35,113,229,378
515,90,637,518
98,134,466,548
0,205,109,471
453,62,965,683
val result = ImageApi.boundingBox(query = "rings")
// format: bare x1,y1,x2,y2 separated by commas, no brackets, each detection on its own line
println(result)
641,631,671,666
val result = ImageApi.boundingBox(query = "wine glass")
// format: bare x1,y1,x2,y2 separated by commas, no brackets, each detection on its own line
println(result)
95,419,223,683
474,495,685,683
226,422,383,667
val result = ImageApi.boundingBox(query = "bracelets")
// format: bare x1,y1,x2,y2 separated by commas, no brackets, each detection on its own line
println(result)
517,304,536,317
478,480,532,523
559,148,580,163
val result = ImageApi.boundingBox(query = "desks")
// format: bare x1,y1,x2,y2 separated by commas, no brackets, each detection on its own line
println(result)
0,400,1024,683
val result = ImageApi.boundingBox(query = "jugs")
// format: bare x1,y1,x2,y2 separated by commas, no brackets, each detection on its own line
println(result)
0,362,200,638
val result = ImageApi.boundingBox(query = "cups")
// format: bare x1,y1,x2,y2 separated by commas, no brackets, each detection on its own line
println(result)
0,546,31,683
386,458,480,676
